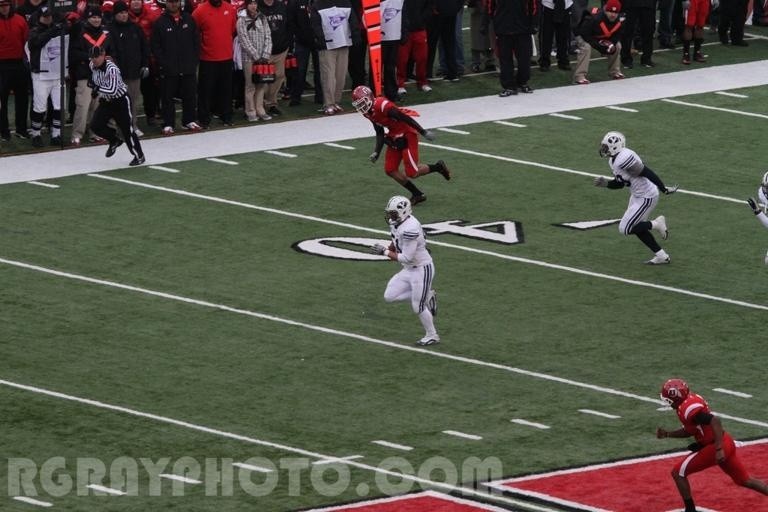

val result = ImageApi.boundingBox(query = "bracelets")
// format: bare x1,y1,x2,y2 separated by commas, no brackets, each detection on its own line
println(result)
664,187,668,195
384,250,389,256
753,209,762,217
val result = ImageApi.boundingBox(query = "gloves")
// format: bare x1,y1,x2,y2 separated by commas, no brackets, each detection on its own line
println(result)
592,176,608,188
91,85,98,98
746,198,759,213
368,152,379,162
371,243,386,255
87,80,94,88
665,183,679,194
140,66,150,79
425,131,434,141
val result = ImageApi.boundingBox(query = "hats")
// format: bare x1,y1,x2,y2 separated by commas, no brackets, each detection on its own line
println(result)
605,0,621,12
112,1,128,14
86,6,101,18
87,46,104,58
39,6,53,17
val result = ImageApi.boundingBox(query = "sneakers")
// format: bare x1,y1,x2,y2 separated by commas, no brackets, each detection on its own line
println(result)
2,128,107,148
324,107,334,115
397,75,459,95
129,155,145,166
682,54,690,63
428,289,438,316
410,193,426,204
644,253,670,264
436,159,449,180
693,53,704,61
416,335,440,346
106,138,123,157
248,105,280,121
334,104,344,113
133,117,201,137
572,79,590,84
612,73,623,79
656,215,667,240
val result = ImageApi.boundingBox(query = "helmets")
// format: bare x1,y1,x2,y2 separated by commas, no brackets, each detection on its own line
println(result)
599,131,625,158
660,378,689,406
351,85,373,115
383,194,412,226
761,172,767,200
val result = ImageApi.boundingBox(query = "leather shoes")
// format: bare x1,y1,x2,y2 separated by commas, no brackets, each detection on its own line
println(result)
540,63,549,72
499,88,517,96
518,84,533,94
558,63,572,70
471,63,479,72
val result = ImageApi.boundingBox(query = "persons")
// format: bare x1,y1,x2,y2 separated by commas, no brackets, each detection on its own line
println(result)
370,194,442,349
588,132,682,268
652,375,768,511
747,171,767,263
2,0,764,169
349,83,453,204
